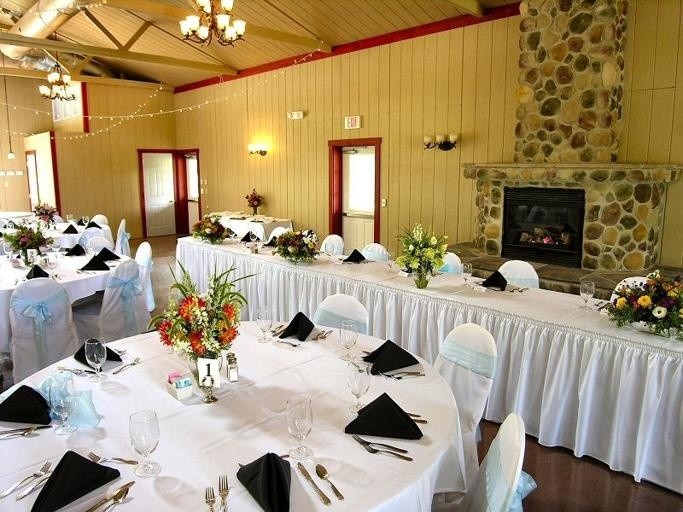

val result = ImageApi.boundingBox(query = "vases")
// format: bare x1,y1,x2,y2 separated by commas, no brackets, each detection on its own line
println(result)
253,207,258,216
413,270,429,288
43,219,51,230
191,350,220,383
23,252,34,264
210,238,222,244
288,255,302,262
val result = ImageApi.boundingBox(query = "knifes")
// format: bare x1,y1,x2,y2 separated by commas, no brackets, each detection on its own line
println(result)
0,425,53,435
298,462,330,505
273,328,286,337
86,481,135,512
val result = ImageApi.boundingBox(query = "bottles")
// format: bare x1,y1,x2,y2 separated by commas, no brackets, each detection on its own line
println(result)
226,353,235,377
230,367,237,382
253,244,258,253
228,357,238,378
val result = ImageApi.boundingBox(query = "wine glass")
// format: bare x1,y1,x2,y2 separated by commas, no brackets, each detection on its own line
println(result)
386,256,394,273
346,361,373,414
286,396,313,460
580,280,594,311
229,229,235,244
256,305,272,343
462,263,472,286
129,410,160,478
340,319,358,361
85,336,107,382
250,230,257,244
47,254,59,280
3,241,14,261
325,242,334,262
49,379,77,435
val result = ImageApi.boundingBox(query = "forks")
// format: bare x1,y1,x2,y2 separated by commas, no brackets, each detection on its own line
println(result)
277,340,300,347
352,433,408,454
88,452,138,465
362,441,413,461
218,474,229,512
312,330,325,341
394,374,425,379
319,330,332,339
378,370,420,377
0,461,52,499
205,486,216,512
113,357,140,374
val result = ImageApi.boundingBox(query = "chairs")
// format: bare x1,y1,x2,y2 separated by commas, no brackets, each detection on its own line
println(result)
495,261,542,292
319,234,346,258
116,219,126,254
134,240,151,283
269,224,291,243
89,214,110,225
121,235,132,259
86,236,114,252
73,261,147,344
313,294,372,334
433,323,497,436
0,276,79,390
360,244,391,263
434,251,462,276
433,413,526,510
52,215,63,223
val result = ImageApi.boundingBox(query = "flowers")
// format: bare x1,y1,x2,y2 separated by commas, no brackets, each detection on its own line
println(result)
244,188,264,206
192,213,231,242
146,255,261,355
599,271,683,340
395,222,448,274
272,229,317,263
0,217,55,252
33,201,58,222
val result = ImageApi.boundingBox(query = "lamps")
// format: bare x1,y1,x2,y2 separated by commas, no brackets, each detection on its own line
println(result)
247,142,272,156
0,51,17,160
177,0,245,49
38,31,74,103
423,132,460,151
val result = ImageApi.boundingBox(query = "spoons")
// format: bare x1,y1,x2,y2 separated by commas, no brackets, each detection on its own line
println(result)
0,426,33,440
270,325,283,334
102,486,129,512
316,464,344,500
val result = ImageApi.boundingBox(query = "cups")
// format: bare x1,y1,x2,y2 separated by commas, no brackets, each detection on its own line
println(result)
67,214,73,222
82,216,89,226
6,223,14,231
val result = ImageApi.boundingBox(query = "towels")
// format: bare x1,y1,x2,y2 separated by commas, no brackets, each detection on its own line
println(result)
364,340,419,375
17,245,41,260
236,451,292,512
280,312,315,342
344,249,367,264
0,222,18,239
97,247,121,262
78,218,86,224
74,339,122,369
264,235,278,248
346,393,424,441
66,243,86,257
241,231,261,243
483,270,507,291
85,221,103,230
81,256,111,273
26,265,51,279
221,228,239,239
32,452,120,512
63,224,80,235
403,258,431,274
1,386,54,425
